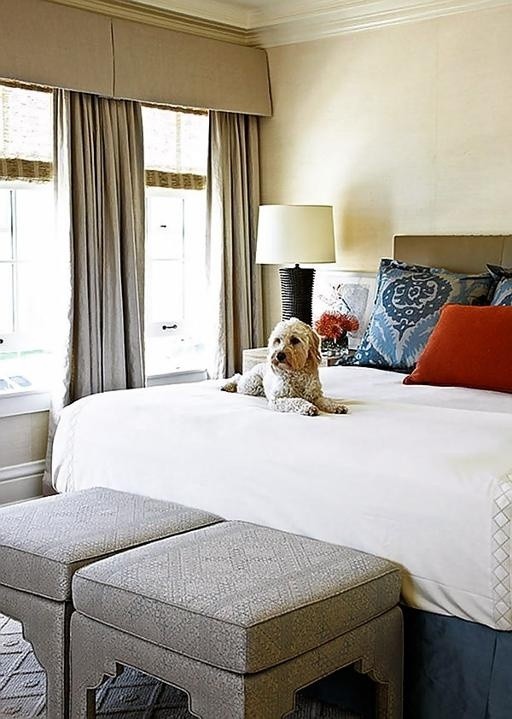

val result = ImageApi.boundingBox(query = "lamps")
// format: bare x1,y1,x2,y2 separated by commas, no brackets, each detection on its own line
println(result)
255,205,336,327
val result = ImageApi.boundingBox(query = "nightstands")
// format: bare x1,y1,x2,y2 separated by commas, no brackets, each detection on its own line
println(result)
242,347,357,377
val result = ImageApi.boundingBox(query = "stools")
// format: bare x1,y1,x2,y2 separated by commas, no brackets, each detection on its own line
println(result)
68,519,405,717
0,487,222,719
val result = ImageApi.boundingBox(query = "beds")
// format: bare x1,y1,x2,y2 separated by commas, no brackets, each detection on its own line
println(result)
51,235,512,719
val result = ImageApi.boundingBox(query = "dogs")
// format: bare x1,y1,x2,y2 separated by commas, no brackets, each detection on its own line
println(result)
218,316,349,417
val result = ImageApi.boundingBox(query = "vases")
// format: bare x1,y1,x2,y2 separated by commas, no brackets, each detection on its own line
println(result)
321,337,348,356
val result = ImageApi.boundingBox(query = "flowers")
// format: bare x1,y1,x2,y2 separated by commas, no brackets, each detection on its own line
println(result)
315,311,359,342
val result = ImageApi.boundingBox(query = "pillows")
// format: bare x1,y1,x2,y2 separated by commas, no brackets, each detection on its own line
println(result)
484,263,512,305
353,257,495,373
403,304,511,395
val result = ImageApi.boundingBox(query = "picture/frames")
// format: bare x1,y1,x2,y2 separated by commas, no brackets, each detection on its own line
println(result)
311,272,377,350
